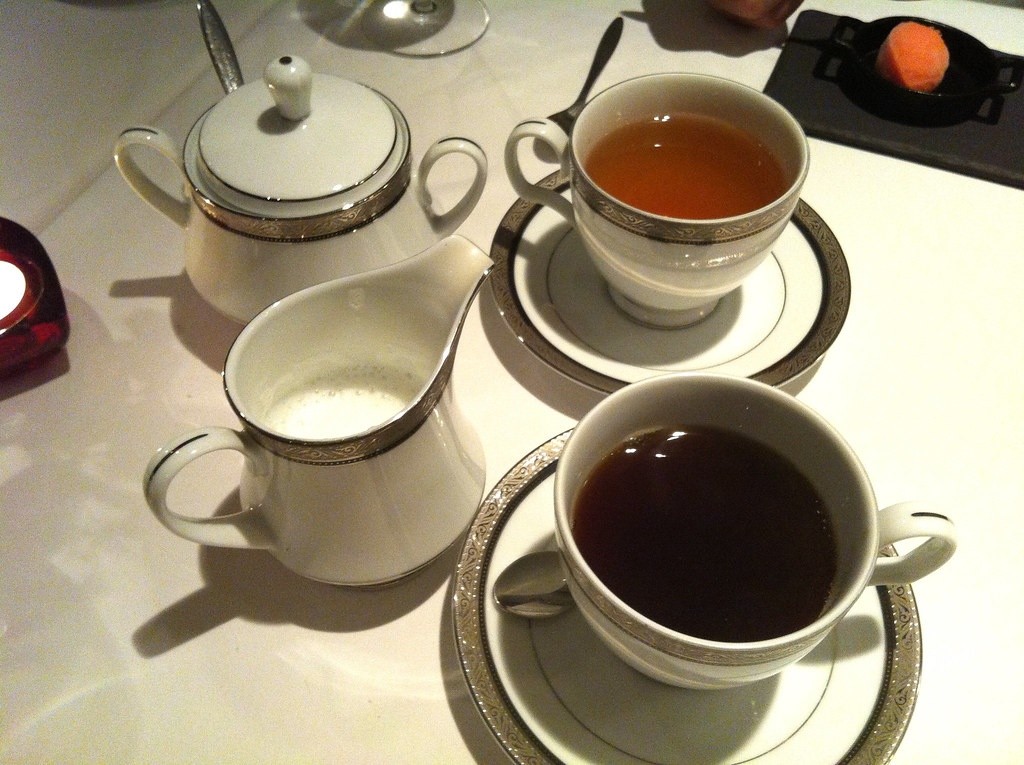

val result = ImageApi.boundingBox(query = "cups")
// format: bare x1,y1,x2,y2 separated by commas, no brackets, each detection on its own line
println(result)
552,374,956,695
111,62,489,330
504,73,809,332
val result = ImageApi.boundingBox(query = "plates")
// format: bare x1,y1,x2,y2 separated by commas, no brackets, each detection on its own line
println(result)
450,425,921,764
477,162,851,398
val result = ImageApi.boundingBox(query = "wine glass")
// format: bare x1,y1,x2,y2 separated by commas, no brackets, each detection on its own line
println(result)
360,0,490,59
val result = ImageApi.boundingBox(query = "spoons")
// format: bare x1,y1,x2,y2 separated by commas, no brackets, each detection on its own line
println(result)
539,17,625,140
494,549,577,622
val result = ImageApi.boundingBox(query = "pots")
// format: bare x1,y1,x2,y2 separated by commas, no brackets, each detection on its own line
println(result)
829,12,1024,120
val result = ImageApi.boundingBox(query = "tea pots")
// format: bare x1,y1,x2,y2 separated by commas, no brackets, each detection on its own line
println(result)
139,236,497,590
